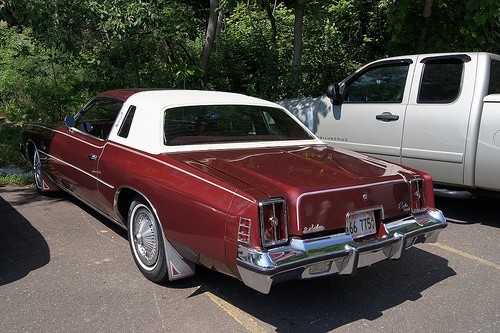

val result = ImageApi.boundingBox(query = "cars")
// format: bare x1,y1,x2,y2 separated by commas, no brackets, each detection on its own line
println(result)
18,88,448,298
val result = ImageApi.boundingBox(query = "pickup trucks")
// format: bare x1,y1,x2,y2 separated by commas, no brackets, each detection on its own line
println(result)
264,51,500,202
